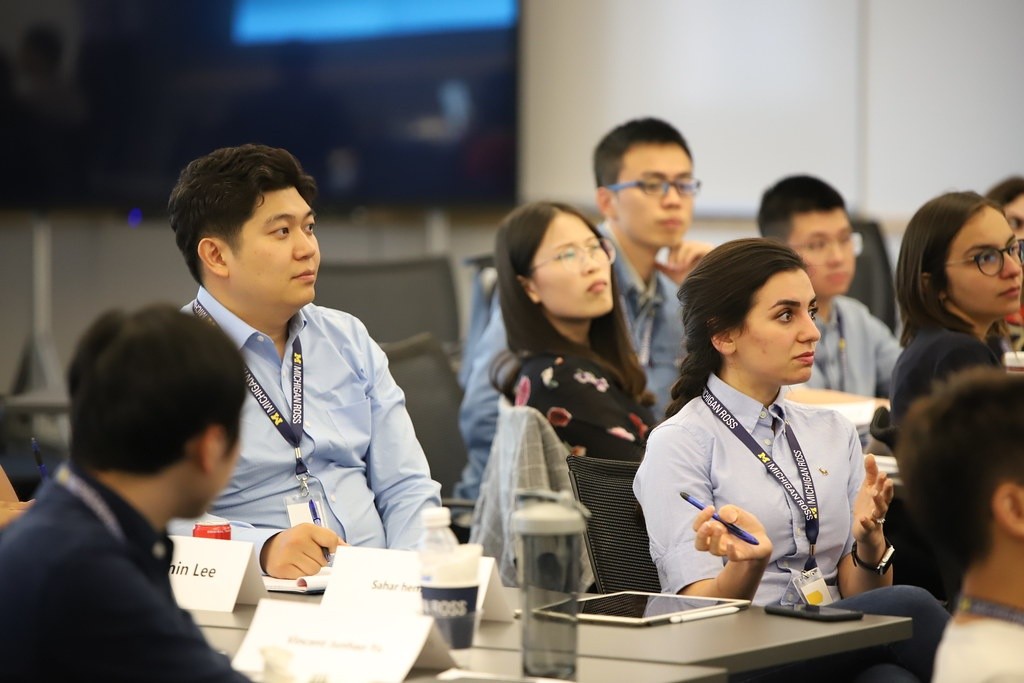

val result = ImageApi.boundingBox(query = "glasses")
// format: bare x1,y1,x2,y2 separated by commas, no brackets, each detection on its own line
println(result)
608,176,702,196
944,241,1024,277
791,232,864,262
529,237,616,273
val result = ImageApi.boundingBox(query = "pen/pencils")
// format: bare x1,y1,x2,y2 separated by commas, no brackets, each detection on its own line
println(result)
669,607,740,624
679,491,761,546
308,499,336,562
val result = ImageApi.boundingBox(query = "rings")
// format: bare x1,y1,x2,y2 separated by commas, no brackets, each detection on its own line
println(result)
872,511,886,524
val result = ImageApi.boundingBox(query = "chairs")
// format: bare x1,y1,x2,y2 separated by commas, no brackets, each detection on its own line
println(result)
0,222,892,594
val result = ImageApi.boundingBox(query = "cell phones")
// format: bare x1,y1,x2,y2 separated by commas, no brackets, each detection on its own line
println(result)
765,604,863,623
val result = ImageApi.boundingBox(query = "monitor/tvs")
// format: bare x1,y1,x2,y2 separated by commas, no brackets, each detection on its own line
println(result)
81,1,524,214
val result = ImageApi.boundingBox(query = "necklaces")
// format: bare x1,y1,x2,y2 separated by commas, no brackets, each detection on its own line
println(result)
814,303,845,391
957,591,1023,624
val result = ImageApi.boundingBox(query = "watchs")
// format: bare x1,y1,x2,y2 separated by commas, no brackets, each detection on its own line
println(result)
851,534,895,576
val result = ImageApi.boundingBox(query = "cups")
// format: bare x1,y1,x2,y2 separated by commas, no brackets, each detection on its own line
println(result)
417,544,484,648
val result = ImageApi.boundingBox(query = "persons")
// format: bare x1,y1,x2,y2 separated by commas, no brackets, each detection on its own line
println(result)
0,303,249,683
169,144,443,580
455,117,1024,683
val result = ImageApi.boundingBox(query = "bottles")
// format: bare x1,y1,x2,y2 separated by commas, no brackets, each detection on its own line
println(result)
1002,349,1024,373
510,501,586,683
416,506,458,544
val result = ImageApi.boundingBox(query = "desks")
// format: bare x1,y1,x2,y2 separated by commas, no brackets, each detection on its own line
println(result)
186,585,913,683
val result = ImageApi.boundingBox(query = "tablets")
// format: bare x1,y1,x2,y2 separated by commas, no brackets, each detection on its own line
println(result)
536,590,751,625
